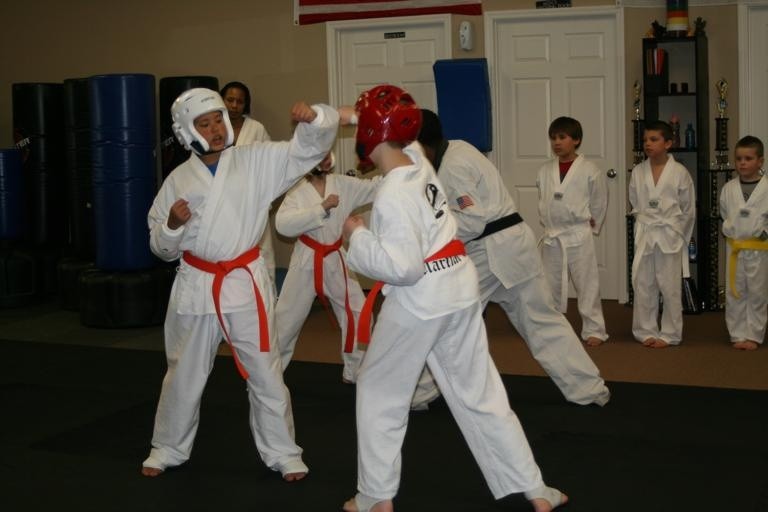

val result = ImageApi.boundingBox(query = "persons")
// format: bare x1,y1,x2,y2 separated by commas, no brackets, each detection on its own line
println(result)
274,152,385,384
140,89,341,482
719,136,768,350
340,84,569,512
219,80,272,142
627,121,697,348
409,109,611,410
536,116,610,347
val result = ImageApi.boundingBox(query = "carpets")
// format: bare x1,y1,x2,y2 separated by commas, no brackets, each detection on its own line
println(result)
0,341,767,512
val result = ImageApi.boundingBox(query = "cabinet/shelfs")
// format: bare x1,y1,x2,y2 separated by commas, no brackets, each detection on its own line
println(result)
627,17,736,316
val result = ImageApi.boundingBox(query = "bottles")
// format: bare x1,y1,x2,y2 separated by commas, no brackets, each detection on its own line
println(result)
668,112,680,148
688,237,697,262
685,123,695,149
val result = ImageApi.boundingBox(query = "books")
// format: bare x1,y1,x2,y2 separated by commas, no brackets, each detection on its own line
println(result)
647,48,669,82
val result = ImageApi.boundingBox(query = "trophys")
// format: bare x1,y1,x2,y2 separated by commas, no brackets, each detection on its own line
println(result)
715,78,730,164
633,80,644,152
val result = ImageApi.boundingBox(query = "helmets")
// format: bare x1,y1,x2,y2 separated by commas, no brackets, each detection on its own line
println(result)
353,84,422,168
171,88,235,155
